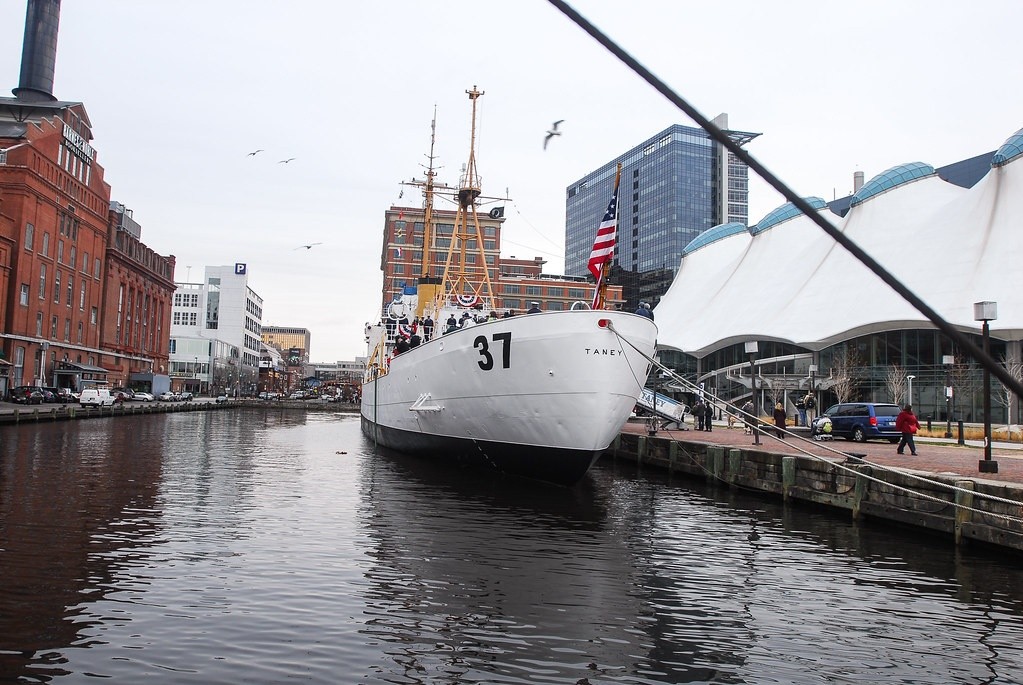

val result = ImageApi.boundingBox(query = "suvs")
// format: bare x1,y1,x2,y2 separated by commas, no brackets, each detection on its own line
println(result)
79,389,116,408
811,403,904,444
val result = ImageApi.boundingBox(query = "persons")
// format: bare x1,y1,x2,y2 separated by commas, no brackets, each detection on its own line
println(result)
742,400,754,435
705,402,713,432
726,400,735,429
614,302,621,310
810,413,832,434
693,400,706,431
774,403,786,439
796,395,807,427
636,301,655,322
336,391,362,404
895,404,921,455
263,385,323,399
804,393,817,427
444,302,542,329
394,316,434,354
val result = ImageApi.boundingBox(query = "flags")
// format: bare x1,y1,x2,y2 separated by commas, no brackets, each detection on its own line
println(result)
588,189,618,311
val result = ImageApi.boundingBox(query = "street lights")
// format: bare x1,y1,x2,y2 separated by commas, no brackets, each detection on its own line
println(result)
809,364,818,395
973,301,999,474
943,355,954,437
745,340,763,446
907,375,916,405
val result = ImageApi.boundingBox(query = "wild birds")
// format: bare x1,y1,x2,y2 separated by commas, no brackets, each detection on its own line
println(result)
248,150,265,155
294,242,323,250
278,158,296,164
543,119,565,151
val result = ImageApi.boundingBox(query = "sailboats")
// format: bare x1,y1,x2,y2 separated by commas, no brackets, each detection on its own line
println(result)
360,85,658,487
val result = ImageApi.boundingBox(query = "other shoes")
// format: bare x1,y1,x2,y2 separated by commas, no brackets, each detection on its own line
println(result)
705,430,708,431
783,437,784,438
897,451,904,454
911,452,918,455
710,430,712,432
778,437,781,439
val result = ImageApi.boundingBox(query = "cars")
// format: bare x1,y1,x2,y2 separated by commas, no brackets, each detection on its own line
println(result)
326,395,334,402
11,385,81,405
216,396,228,403
109,387,155,402
259,390,304,399
159,391,193,402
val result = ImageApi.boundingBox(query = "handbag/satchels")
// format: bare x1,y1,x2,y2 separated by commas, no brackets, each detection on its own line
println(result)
730,416,736,423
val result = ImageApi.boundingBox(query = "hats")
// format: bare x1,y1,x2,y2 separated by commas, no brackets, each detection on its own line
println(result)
415,315,418,317
638,302,645,306
421,316,425,318
451,314,455,316
427,315,430,317
531,302,539,305
